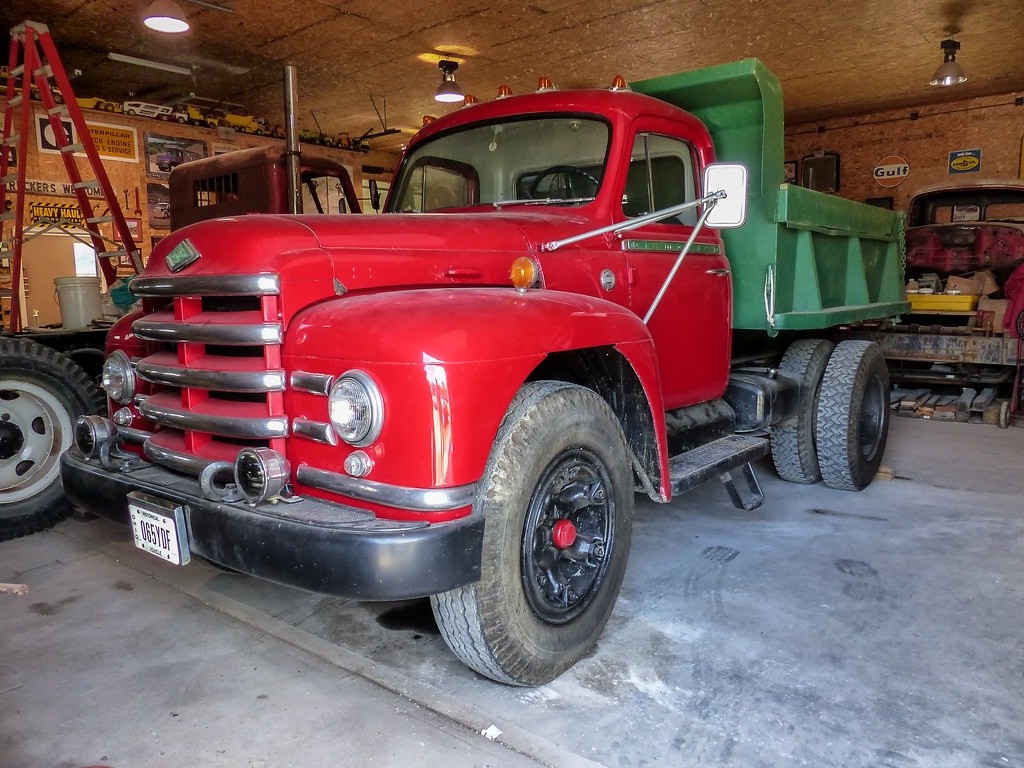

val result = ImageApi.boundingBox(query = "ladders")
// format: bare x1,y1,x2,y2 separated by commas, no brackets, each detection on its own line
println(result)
1,20,150,335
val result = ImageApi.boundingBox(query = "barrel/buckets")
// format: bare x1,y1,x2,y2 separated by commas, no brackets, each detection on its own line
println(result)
53,277,103,329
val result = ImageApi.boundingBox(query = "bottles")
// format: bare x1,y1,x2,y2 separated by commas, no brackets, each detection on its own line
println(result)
906,279,918,294
919,288,933,295
947,290,961,295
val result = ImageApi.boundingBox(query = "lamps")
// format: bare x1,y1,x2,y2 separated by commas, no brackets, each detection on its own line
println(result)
108,50,191,76
930,39,968,86
434,59,464,102
144,0,190,34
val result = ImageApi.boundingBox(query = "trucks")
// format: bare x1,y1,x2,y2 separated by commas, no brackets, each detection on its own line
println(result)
56,56,917,687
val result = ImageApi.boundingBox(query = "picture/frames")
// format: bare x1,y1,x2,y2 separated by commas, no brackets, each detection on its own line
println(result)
143,131,207,180
783,161,798,184
119,246,141,268
151,235,166,249
111,217,143,242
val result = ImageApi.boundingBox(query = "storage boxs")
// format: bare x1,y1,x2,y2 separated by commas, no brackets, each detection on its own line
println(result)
907,293,981,312
944,274,982,295
978,296,1009,333
968,270,1001,295
